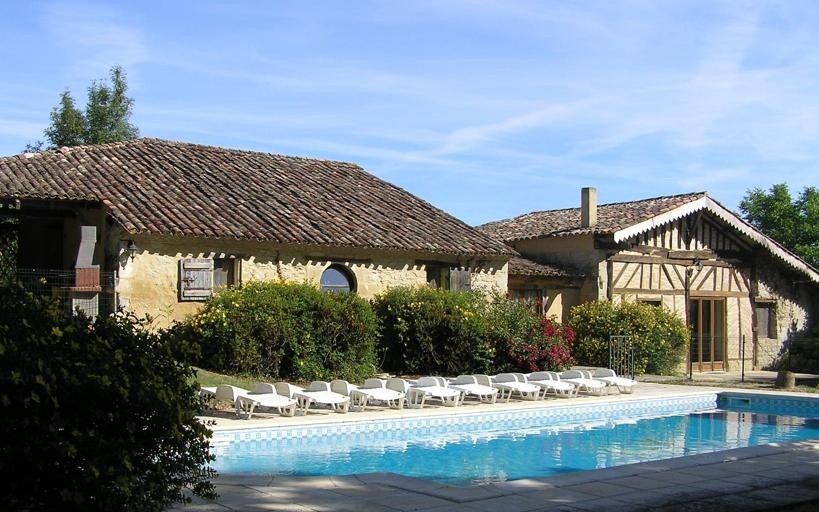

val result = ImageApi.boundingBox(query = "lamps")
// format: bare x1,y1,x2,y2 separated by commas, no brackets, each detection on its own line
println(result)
685,255,704,276
120,237,138,265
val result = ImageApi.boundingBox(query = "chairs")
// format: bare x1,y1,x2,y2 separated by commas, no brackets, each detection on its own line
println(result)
196,365,637,421
